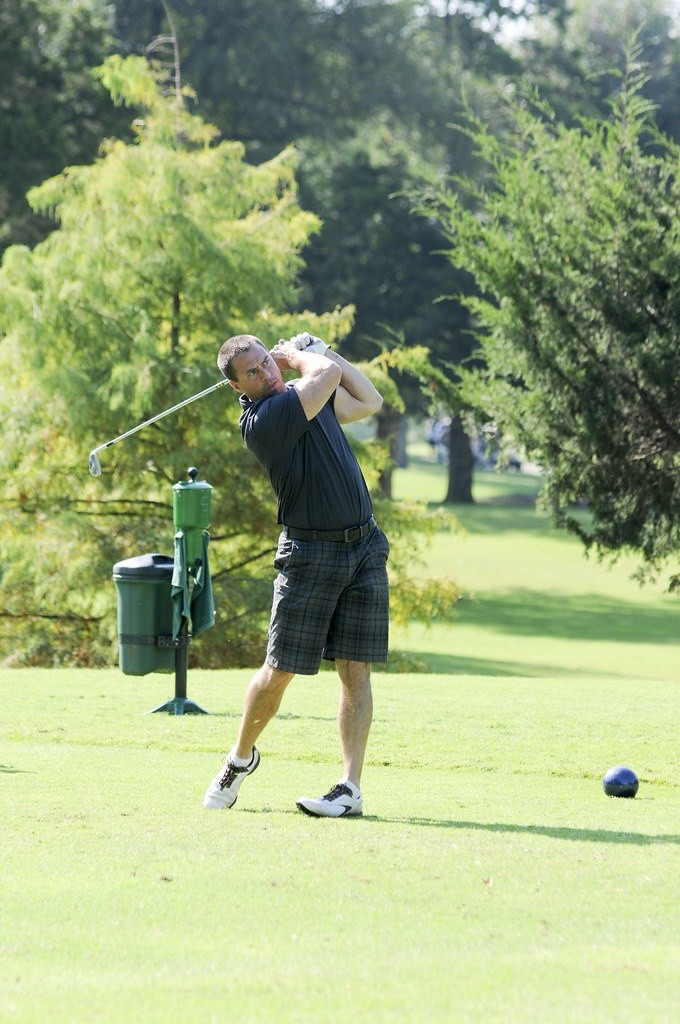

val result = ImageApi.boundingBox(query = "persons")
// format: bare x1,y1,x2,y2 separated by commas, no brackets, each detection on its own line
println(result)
202,331,391,817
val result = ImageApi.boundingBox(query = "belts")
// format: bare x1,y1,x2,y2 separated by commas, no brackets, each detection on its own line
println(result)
283,516,376,543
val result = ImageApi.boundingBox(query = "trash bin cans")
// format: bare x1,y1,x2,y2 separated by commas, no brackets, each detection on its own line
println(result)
112,552,177,675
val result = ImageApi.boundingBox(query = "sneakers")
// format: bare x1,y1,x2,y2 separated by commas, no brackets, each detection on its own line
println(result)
203,745,260,810
295,783,364,817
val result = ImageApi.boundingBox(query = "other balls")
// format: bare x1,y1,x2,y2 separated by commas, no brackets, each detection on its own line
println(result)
602,766,639,798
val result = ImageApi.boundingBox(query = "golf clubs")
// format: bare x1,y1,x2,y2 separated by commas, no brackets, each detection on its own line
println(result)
88,378,230,477
